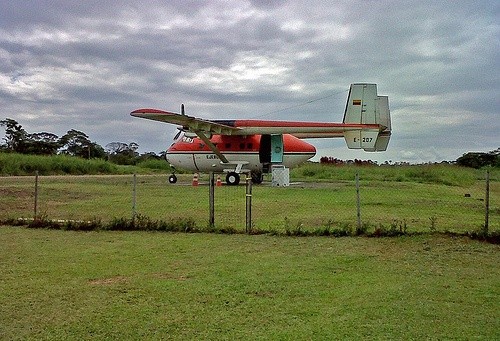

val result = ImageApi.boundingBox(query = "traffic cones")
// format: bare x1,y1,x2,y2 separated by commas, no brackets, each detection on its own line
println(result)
192,173,198,187
216,174,221,187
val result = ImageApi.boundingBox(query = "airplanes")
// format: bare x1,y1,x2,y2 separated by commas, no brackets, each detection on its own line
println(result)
129,83,392,184
